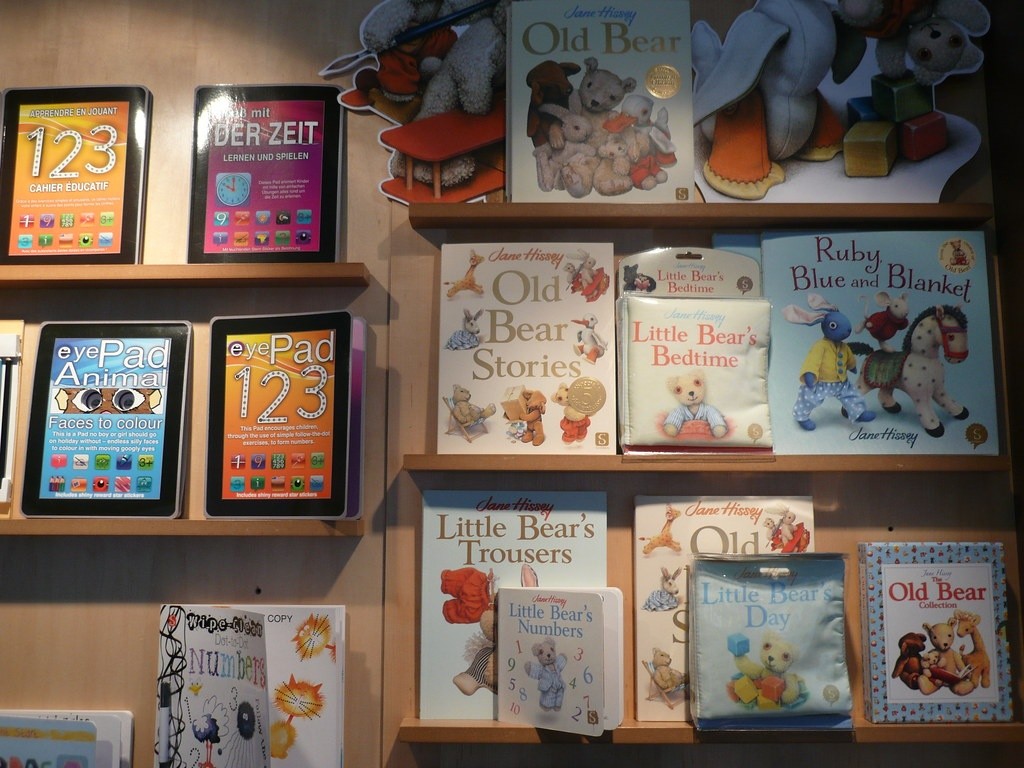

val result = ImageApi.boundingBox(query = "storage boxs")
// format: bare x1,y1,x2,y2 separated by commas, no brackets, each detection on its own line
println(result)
858,541,1015,723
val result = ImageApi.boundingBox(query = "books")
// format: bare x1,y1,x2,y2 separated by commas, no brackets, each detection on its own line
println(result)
437,243,616,455
420,491,607,719
632,495,815,722
761,228,999,455
0,708,135,768
505,0,694,204
498,587,624,736
153,603,346,768
859,540,1015,724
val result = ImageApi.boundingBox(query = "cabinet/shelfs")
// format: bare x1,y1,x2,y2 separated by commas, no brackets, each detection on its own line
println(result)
0,262,372,536
392,204,1024,743
